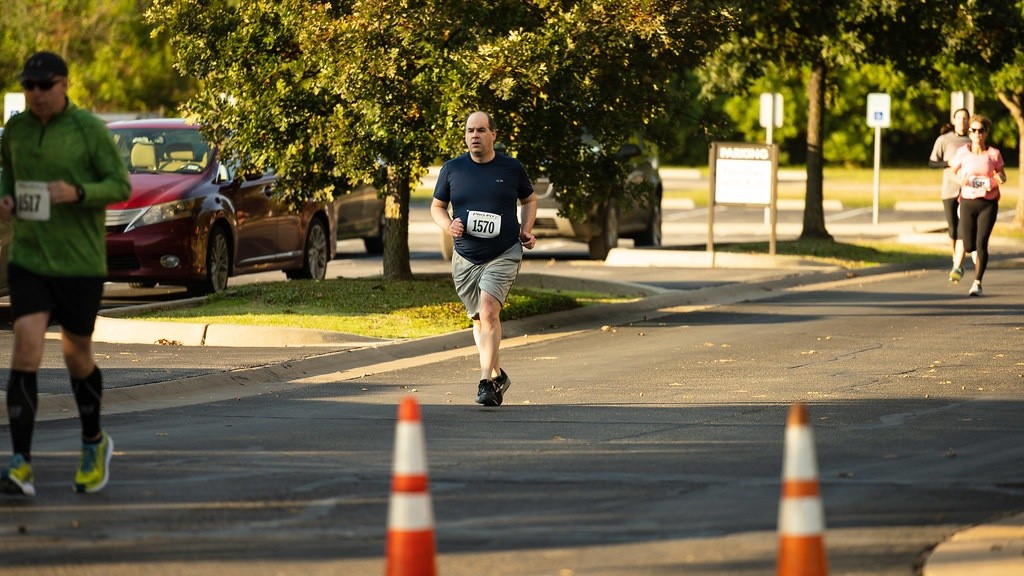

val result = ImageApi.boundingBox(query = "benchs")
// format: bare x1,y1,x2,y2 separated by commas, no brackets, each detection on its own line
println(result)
158,143,206,173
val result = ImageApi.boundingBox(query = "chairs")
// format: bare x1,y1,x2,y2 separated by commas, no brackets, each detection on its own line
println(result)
131,143,158,173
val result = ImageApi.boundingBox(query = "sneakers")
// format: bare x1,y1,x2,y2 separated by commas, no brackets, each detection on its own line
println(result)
74,430,114,494
0,454,36,497
484,367,511,407
476,380,494,403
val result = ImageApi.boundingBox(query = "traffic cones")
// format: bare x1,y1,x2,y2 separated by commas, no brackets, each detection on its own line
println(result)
385,394,439,576
776,401,828,576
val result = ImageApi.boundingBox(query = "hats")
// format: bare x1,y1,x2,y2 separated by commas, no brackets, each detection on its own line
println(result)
18,51,68,83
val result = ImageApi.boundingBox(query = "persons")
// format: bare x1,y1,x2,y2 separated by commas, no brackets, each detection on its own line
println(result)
928,108,970,285
429,112,537,406
953,115,1007,296
0,51,133,500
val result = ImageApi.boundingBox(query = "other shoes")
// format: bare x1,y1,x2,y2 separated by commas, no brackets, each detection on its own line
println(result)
949,265,964,283
972,250,977,265
969,279,981,295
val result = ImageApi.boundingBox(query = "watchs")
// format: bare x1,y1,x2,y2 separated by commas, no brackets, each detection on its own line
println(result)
76,184,85,201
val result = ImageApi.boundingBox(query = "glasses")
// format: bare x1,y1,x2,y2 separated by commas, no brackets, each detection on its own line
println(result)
22,79,63,91
969,128,987,133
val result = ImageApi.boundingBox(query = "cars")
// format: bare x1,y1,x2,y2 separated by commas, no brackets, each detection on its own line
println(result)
440,128,664,262
93,117,340,295
336,144,389,258
